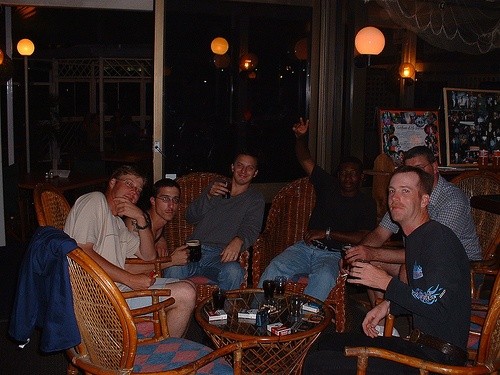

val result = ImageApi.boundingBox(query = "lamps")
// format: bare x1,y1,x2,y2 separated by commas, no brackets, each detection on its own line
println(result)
210,37,229,55
295,38,308,60
354,26,385,66
399,63,415,87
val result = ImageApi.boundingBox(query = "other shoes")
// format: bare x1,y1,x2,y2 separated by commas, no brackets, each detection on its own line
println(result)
298,321,312,332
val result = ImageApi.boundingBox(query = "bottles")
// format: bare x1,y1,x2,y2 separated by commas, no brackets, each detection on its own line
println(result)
479,142,488,168
492,146,500,168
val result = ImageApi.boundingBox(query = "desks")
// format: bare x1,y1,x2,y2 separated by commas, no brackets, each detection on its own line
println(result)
17,175,107,241
195,288,332,375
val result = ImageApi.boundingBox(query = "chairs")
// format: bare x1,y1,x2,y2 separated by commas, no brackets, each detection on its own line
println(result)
34,169,500,375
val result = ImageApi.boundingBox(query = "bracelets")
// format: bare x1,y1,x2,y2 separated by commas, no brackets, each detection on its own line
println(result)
325,227,332,240
136,218,149,230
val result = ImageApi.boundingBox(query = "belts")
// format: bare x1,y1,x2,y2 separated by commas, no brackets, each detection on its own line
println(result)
311,241,341,252
408,328,465,359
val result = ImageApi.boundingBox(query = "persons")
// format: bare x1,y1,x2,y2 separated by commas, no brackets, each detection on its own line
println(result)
124,177,189,275
63,162,196,338
251,117,377,308
339,145,485,306
301,164,473,375
162,150,265,290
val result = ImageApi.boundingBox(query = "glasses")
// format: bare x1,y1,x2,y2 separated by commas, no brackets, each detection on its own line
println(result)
156,196,180,203
419,159,434,171
115,177,142,196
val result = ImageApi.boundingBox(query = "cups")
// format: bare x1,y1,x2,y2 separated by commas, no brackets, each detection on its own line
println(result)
356,259,369,286
222,177,232,199
341,243,354,269
263,276,304,316
212,287,226,308
186,240,201,261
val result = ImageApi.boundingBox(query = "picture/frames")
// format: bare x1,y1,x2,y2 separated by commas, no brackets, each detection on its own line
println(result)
376,107,443,167
443,87,500,167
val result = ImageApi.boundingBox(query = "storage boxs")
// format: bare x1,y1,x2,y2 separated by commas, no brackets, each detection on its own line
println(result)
303,303,321,313
267,322,291,336
238,309,258,319
